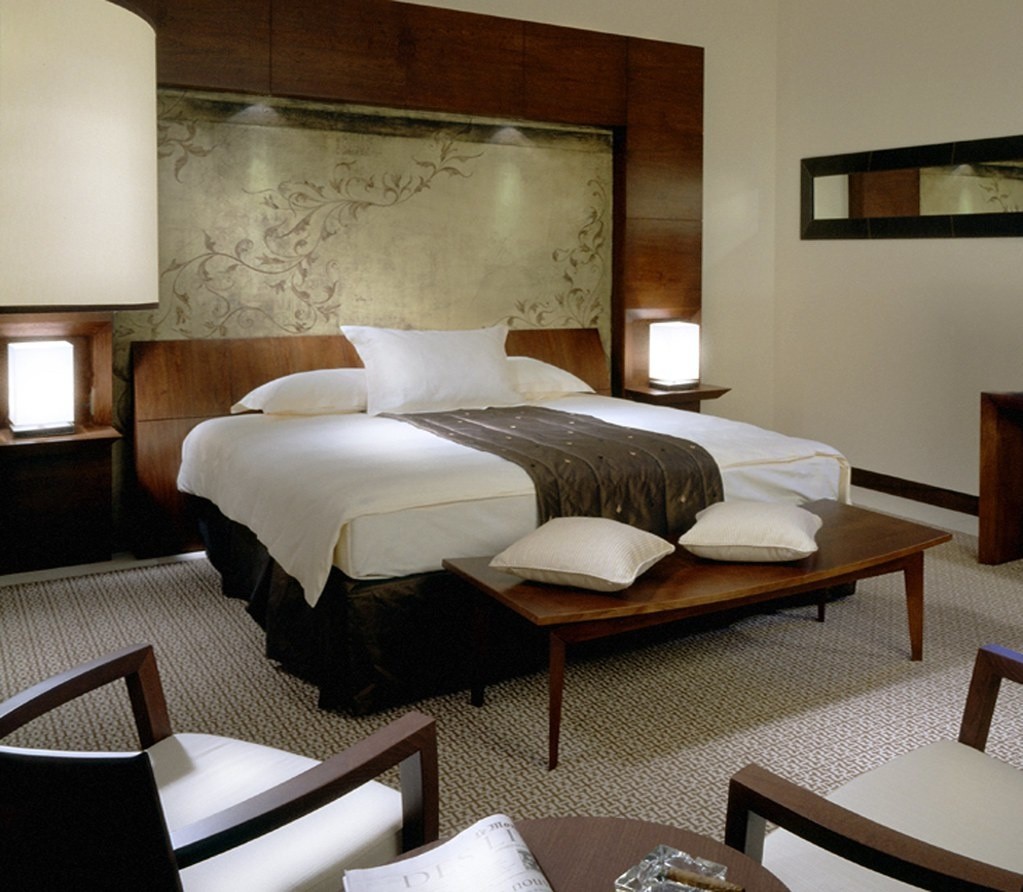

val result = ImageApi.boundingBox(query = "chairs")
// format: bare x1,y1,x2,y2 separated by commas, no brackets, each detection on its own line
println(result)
0,644,438,892
724,645,1023,892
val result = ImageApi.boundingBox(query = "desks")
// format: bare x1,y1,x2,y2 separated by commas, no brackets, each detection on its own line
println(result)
443,497,955,772
978,391,1023,566
391,817,790,892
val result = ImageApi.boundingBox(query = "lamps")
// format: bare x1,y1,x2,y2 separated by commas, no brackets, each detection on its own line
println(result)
0,0,160,315
8,340,75,436
648,321,700,392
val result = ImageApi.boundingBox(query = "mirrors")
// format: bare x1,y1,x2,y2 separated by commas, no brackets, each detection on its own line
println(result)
799,135,1023,238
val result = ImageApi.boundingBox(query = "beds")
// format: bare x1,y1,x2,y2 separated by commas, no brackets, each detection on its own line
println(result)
132,326,858,718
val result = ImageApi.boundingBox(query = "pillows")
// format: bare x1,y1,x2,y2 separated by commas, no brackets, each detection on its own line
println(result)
487,517,676,592
506,356,596,402
678,502,823,562
340,323,528,417
230,367,368,416
